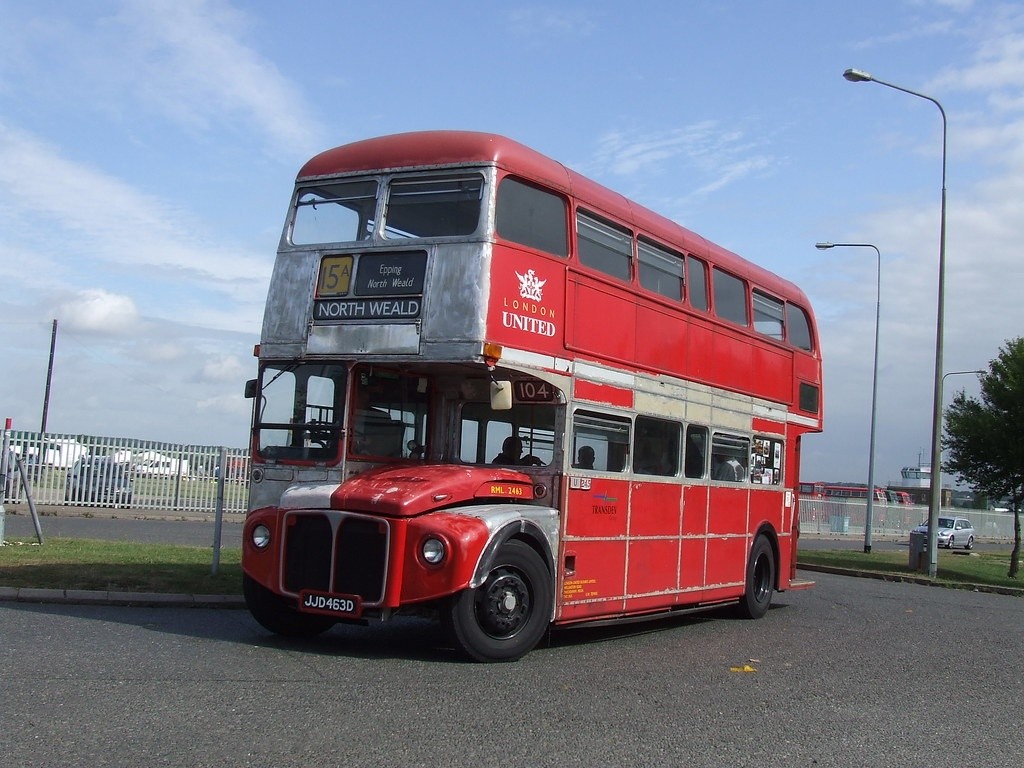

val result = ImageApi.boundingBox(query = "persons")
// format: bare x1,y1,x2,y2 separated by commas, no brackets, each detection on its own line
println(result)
570,445,596,470
491,436,547,467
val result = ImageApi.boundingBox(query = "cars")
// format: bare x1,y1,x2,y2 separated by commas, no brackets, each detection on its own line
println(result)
1,450,25,499
913,518,975,550
64,459,134,508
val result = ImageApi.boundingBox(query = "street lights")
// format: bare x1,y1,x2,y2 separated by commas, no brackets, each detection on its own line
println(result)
843,67,988,579
814,241,881,554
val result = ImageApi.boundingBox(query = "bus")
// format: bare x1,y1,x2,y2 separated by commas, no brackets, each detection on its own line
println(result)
799,481,912,506
240,131,824,665
